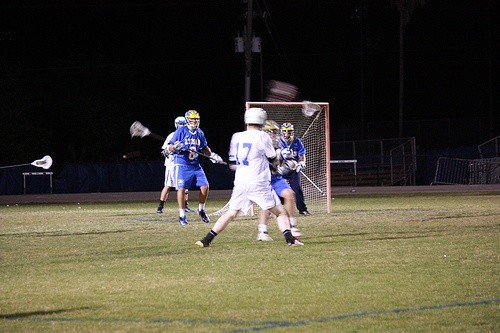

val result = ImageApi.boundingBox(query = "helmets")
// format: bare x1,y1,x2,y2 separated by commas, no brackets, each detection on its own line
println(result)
185,110,201,130
260,119,280,138
244,108,267,124
174,117,186,129
281,123,295,141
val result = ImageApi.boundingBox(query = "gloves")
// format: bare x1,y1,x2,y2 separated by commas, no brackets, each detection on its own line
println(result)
277,148,306,176
211,152,223,164
173,141,184,153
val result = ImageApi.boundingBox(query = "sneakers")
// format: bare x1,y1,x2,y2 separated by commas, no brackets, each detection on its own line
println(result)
157,206,162,213
290,228,301,237
299,209,313,215
195,239,210,248
288,217,297,226
185,206,195,212
258,232,274,241
197,209,210,223
178,216,188,225
286,236,304,246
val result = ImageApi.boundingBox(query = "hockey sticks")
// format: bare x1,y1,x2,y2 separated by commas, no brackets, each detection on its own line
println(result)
130,121,227,165
299,170,325,196
281,100,321,167
0,155,53,169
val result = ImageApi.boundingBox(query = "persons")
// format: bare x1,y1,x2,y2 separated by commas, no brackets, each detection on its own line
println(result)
166,109,223,225
193,106,304,249
154,115,197,216
278,122,313,218
257,120,303,242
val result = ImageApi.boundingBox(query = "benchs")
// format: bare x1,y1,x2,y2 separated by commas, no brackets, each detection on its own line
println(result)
328,164,415,186
